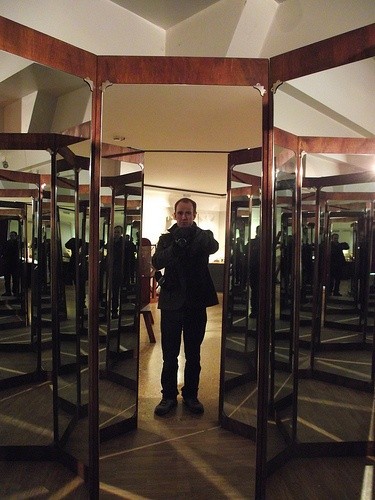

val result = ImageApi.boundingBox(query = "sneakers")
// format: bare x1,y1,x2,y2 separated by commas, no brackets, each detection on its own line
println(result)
2,292,11,296
112,311,119,319
334,292,342,296
182,398,204,413
249,312,257,319
155,398,177,416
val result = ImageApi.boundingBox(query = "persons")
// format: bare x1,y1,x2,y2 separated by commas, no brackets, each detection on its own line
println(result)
6,225,364,330
152,198,220,416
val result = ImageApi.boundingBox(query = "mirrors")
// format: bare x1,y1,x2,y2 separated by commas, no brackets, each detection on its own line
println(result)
0,13,375,500
